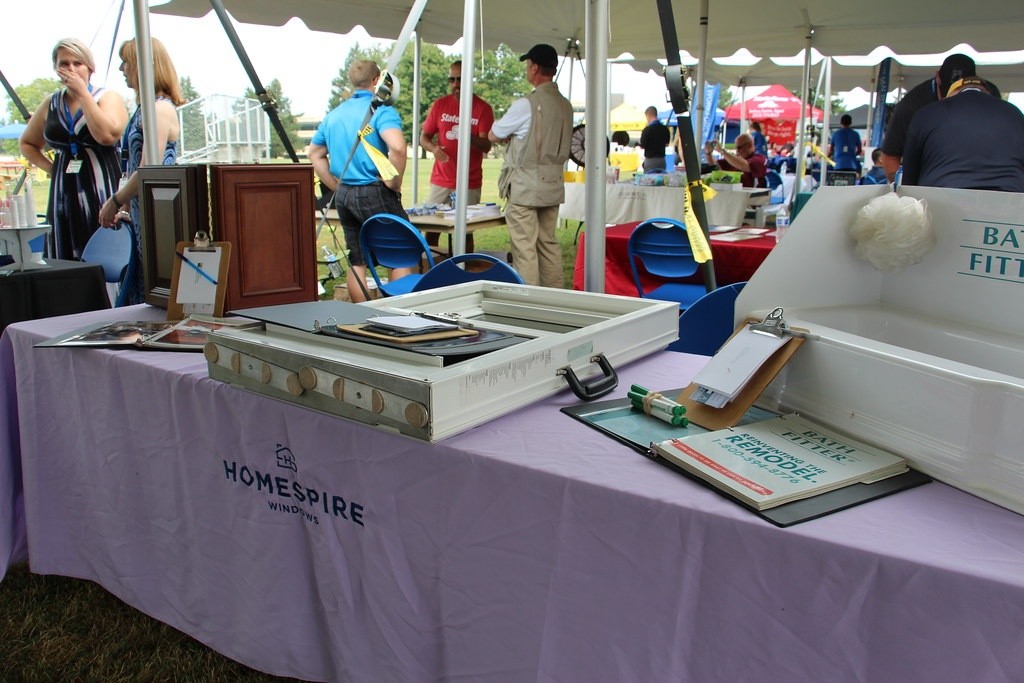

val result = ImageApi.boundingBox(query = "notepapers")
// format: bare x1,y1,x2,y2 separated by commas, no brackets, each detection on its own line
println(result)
477,202,496,206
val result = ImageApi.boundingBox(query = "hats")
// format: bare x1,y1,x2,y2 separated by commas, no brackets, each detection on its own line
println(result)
945,76,1001,98
520,44,558,67
939,54,976,98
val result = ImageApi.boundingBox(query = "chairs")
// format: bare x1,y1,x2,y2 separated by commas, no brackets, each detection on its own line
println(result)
628,217,734,312
80,220,137,309
609,153,639,182
664,281,747,356
765,172,784,204
360,213,526,298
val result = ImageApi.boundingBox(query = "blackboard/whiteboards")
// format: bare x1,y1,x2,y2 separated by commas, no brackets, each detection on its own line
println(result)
828,171,857,186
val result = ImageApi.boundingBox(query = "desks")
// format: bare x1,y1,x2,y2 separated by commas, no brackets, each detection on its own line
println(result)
1,301,1022,683
406,203,507,271
761,172,818,203
0,256,112,334
574,220,777,303
555,182,772,229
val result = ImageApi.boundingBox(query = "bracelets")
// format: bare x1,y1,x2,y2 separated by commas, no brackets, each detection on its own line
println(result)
118,211,128,215
112,194,123,209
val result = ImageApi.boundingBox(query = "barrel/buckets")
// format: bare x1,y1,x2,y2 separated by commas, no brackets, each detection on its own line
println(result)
665,151,677,173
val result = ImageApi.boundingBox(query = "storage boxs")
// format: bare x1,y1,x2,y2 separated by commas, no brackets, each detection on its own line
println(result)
467,249,509,271
410,246,450,275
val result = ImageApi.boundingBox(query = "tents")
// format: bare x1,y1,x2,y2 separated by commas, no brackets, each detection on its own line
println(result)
609,80,825,147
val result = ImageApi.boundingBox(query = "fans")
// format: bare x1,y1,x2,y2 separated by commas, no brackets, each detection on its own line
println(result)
570,124,610,168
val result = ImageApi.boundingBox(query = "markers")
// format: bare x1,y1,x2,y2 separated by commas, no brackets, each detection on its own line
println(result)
631,400,680,425
627,392,680,415
680,416,689,428
631,384,686,415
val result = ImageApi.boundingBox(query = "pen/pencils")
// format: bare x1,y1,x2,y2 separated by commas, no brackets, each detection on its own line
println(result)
747,321,820,341
415,312,458,324
894,166,904,193
175,251,218,285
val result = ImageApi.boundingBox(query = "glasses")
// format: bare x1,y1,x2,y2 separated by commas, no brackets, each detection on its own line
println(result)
737,143,749,150
447,76,461,83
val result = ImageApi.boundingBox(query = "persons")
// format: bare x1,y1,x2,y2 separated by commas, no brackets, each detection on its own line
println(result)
18,36,187,310
305,60,412,305
827,114,903,192
704,122,768,188
639,106,671,174
487,44,574,288
419,59,493,254
880,53,1024,194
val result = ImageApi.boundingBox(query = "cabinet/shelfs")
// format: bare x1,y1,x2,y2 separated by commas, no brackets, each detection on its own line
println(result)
137,162,316,313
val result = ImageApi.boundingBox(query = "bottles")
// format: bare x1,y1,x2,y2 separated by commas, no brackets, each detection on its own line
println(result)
322,245,345,280
894,165,903,192
775,202,791,243
780,161,787,176
